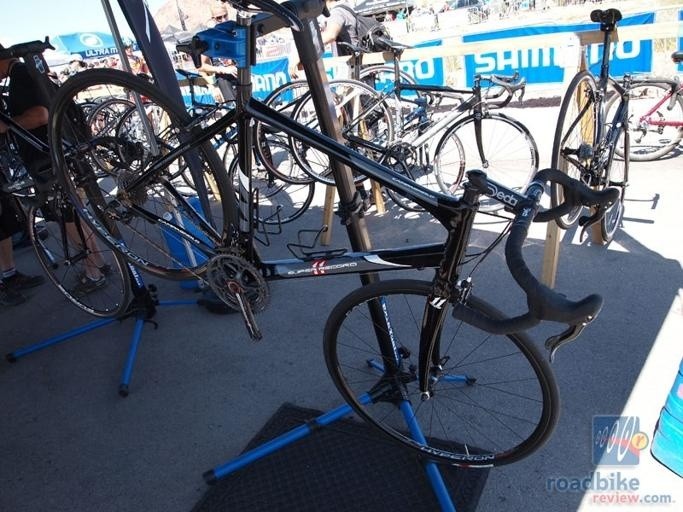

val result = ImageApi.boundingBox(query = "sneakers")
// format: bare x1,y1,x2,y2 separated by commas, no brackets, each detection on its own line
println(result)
67,273,106,298
96,263,113,276
0,278,27,307
2,271,46,290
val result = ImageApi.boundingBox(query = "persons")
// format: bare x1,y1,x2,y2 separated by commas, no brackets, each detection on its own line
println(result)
195,0,254,106
50,36,159,129
291,0,391,217
384,0,536,27
0,43,115,306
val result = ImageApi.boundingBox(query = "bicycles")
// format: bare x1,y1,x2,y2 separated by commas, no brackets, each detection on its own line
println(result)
0,0,683,471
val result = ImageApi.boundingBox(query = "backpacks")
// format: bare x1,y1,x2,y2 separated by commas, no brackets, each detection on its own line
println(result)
43,75,93,147
